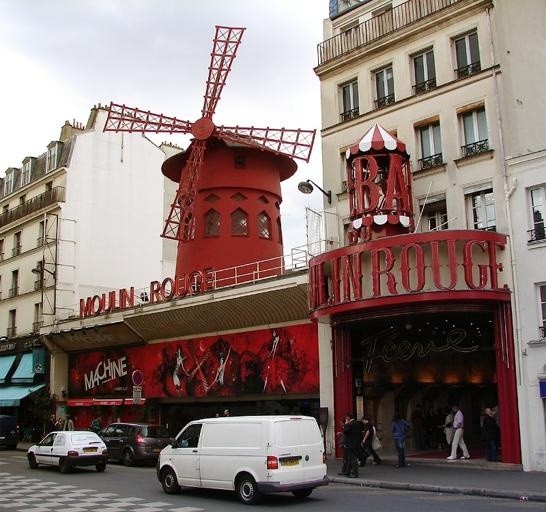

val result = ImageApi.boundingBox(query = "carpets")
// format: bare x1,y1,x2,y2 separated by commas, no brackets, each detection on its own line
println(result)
407,441,487,459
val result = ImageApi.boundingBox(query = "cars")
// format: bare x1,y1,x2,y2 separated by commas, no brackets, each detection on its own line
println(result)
98,421,174,466
27,429,108,473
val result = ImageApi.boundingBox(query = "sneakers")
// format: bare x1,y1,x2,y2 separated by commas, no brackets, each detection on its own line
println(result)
447,456,470,460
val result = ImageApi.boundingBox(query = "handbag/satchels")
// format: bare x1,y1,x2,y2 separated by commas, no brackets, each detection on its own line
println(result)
372,437,381,450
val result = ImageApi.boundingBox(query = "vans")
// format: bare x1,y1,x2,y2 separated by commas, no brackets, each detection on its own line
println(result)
156,415,328,505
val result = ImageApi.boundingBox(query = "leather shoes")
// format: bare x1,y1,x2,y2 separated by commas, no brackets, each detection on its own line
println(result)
338,470,359,478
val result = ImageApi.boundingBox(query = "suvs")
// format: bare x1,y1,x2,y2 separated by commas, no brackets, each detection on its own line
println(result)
0,414,20,448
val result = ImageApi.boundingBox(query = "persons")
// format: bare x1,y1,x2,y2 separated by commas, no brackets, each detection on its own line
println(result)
480,408,501,462
90,415,104,432
215,409,231,418
47,415,74,432
439,404,470,460
337,410,382,477
390,410,412,468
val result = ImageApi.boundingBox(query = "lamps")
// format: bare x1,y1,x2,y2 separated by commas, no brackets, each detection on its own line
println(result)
31,266,55,281
